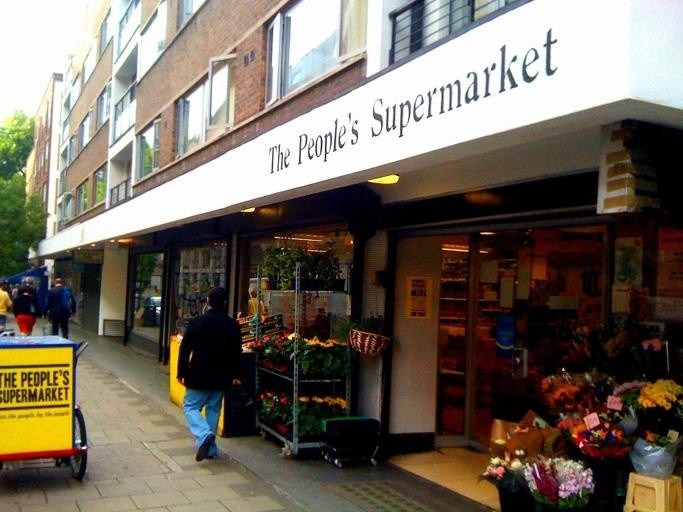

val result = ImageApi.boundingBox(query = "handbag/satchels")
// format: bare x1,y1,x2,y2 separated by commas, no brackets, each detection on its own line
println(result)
30,304,39,317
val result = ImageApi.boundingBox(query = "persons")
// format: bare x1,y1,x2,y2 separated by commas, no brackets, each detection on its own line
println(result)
0,276,42,336
248,283,265,314
43,278,76,339
45,285,55,326
176,286,240,461
315,308,326,329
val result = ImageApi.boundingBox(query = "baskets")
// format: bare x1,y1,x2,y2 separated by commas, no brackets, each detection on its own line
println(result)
349,329,389,357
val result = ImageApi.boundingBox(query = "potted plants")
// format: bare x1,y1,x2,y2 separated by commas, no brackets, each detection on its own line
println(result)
261,240,345,292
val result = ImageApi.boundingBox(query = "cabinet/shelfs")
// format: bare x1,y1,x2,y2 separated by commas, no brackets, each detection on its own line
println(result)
255,263,352,456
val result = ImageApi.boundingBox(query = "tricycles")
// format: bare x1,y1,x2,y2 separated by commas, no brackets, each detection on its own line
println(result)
0,328,88,481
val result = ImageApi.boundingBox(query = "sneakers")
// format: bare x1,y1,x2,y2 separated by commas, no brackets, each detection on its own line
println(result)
195,434,217,461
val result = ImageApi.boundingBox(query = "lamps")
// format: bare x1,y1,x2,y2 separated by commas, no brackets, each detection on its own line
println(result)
368,173,400,185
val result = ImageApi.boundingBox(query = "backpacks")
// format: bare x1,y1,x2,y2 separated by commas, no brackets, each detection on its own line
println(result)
62,287,73,314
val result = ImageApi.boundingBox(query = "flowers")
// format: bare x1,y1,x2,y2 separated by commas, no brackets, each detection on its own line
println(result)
248,329,347,376
258,390,347,438
478,372,682,512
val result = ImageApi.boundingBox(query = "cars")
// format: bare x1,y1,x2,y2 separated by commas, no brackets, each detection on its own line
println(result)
143,297,161,327
